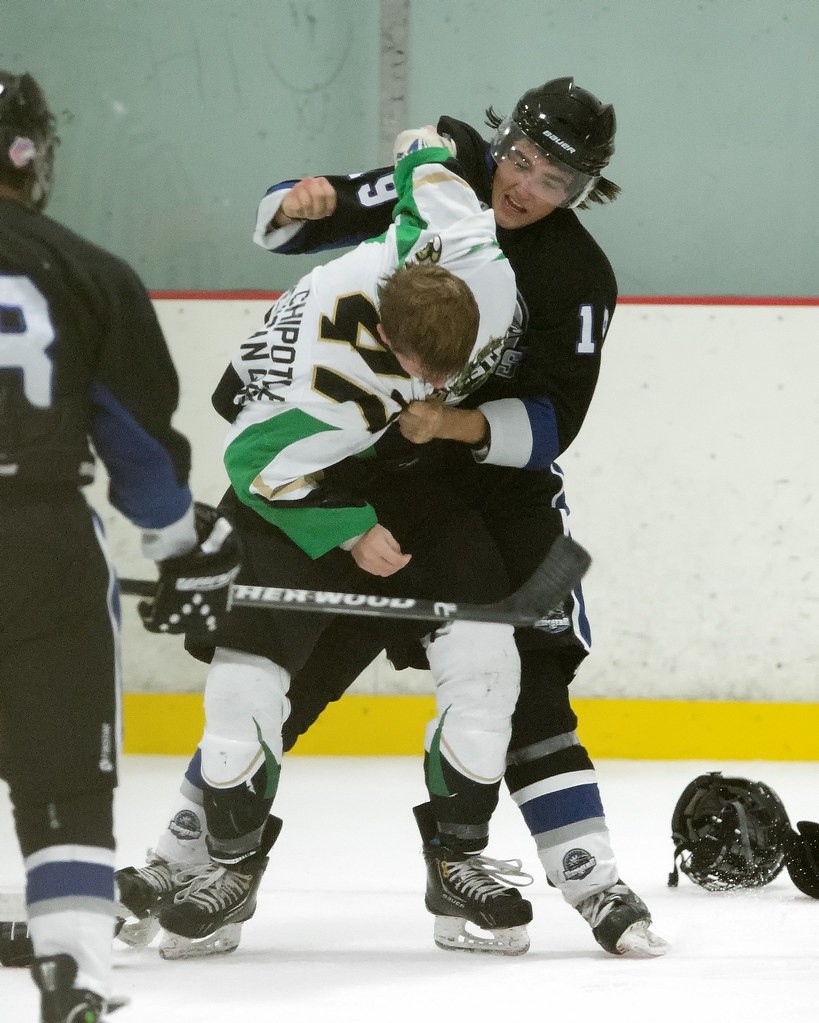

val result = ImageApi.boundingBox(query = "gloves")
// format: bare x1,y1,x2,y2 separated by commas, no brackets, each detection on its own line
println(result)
138,502,239,635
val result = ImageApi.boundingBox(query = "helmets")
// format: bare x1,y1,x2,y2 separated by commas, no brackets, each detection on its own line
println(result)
0,71,58,211
670,772,790,888
506,76,616,177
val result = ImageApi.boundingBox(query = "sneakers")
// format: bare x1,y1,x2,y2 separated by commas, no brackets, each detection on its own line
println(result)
576,880,669,956
159,814,283,961
412,801,534,956
111,856,187,943
30,952,106,1023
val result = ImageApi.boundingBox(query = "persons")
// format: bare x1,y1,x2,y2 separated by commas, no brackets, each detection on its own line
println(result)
0,71,241,1023
113,76,674,960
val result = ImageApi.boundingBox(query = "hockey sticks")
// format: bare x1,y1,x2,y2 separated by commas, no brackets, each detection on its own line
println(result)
117,534,592,627
